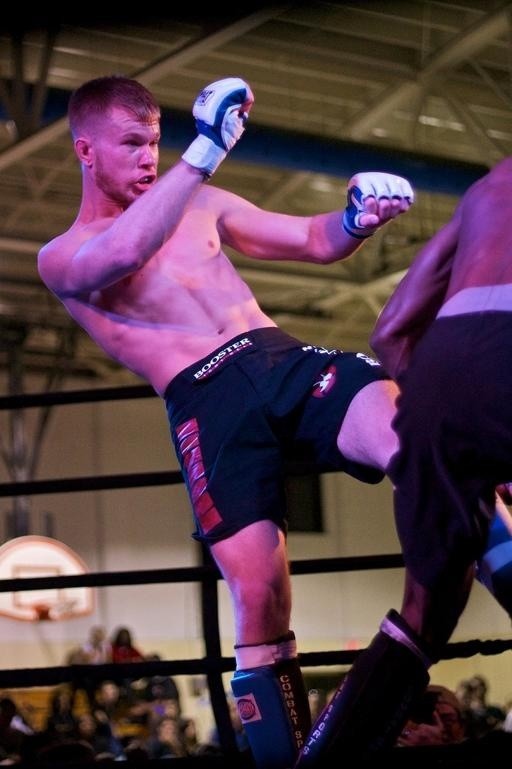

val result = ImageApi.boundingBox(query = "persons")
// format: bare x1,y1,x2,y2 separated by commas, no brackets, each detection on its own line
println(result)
209,675,512,768
37,75,512,769
293,154,512,769
1,625,201,769
